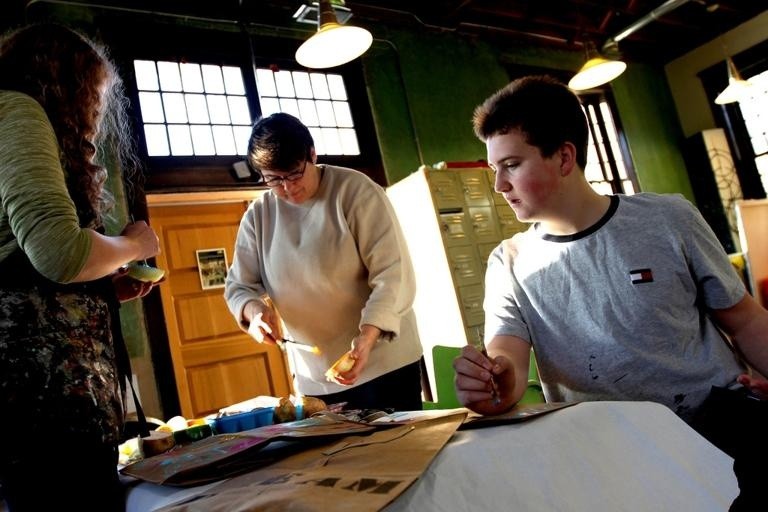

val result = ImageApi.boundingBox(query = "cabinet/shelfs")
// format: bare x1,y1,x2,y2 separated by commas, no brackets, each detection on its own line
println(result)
378,157,539,406
679,126,745,257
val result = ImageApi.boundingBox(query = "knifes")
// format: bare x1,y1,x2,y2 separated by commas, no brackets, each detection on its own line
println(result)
280,338,318,355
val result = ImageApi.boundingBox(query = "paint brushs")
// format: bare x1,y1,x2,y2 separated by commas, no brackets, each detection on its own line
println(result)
284,340,320,353
130,213,150,267
478,333,501,407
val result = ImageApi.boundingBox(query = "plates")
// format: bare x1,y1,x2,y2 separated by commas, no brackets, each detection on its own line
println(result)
125,264,166,282
327,351,354,382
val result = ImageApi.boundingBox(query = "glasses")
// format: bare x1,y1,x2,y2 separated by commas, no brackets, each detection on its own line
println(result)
262,153,309,186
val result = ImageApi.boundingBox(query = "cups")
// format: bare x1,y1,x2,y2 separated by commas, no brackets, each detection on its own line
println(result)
215,404,275,435
137,425,213,461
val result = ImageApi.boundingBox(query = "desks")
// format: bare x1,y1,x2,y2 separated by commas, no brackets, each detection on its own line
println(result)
114,398,741,508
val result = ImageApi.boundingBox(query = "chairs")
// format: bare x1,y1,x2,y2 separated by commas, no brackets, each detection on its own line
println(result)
418,346,542,409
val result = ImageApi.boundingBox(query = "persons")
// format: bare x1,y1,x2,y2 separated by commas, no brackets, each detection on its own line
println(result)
451,75,767,511
0,23,168,511
222,112,425,411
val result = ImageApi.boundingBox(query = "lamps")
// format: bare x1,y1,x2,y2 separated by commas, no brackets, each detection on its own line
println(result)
284,0,377,74
711,27,761,107
565,2,629,94
